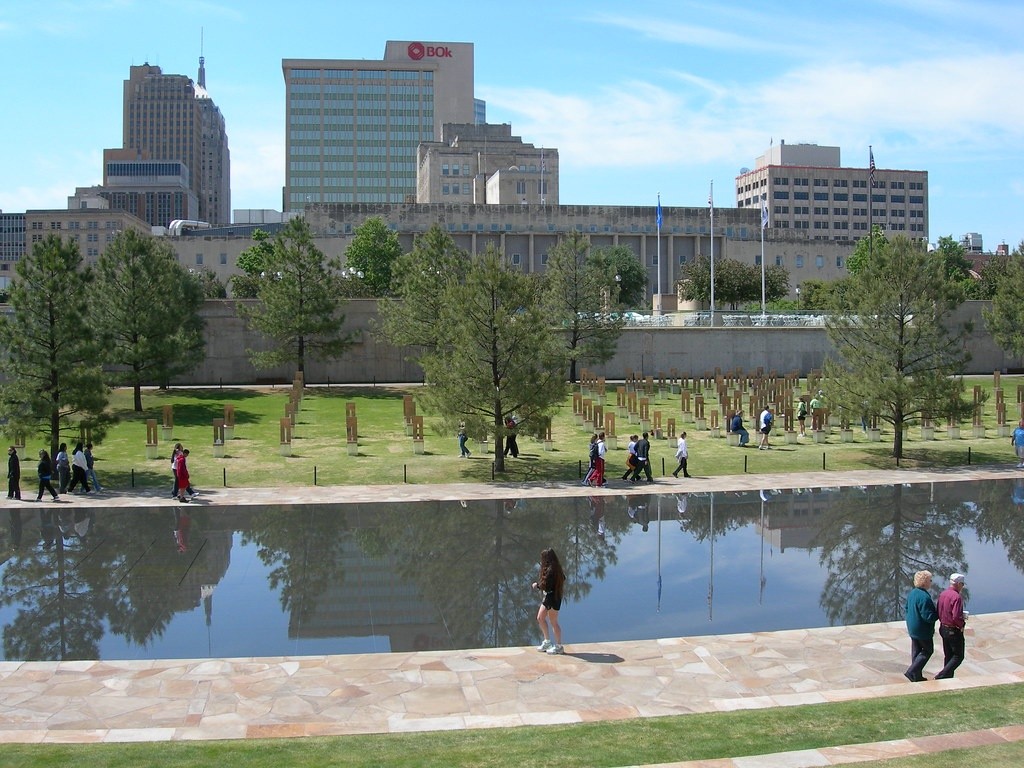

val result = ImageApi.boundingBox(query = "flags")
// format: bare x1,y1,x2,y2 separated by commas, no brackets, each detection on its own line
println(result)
542,150,545,171
657,197,663,228
708,185,715,217
761,200,767,227
870,149,875,186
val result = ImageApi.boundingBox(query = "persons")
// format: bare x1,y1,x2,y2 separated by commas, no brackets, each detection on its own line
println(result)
172,506,191,554
503,417,519,458
531,547,566,655
79,444,103,492
731,410,749,447
176,449,189,503
903,570,966,682
5,445,21,499
580,431,691,487
7,508,94,553
459,433,471,459
171,443,199,500
457,493,689,537
760,405,769,445
56,443,72,494
1011,478,1024,511
1010,418,1024,468
797,389,828,437
34,449,60,501
840,400,871,434
759,409,774,450
67,443,96,494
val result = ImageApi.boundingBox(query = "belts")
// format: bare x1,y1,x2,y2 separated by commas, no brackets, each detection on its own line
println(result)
940,624,960,629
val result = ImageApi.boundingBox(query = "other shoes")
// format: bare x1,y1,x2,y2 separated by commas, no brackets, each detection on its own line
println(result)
621,476,654,485
173,491,199,503
1017,462,1024,468
5,495,21,500
916,674,927,681
904,672,917,682
57,489,109,496
759,445,772,450
580,477,608,488
35,496,60,501
738,443,748,447
672,473,692,478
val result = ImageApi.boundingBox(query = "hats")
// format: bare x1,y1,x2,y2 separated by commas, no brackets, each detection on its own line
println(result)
950,573,967,586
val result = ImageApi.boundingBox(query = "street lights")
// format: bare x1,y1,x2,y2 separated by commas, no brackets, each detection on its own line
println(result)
795,288,801,309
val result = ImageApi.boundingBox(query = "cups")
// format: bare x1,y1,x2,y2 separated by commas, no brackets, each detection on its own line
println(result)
963,611,969,619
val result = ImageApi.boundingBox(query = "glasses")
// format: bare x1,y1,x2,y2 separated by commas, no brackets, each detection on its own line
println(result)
926,579,933,582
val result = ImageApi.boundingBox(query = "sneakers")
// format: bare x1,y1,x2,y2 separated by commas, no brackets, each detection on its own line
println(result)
536,640,552,651
546,644,564,655
